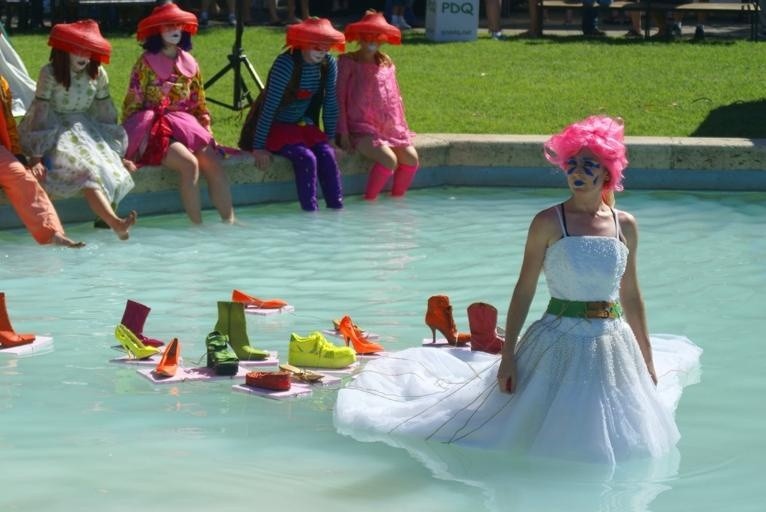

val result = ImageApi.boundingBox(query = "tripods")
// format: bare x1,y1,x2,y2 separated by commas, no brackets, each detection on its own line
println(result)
203,1,264,112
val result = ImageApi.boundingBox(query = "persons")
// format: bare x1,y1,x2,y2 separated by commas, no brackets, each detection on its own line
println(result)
0,73,86,248
672,0,709,43
0,1,413,37
337,9,419,201
16,20,137,240
582,0,612,37
237,17,346,212
334,112,706,465
121,5,241,225
624,11,681,40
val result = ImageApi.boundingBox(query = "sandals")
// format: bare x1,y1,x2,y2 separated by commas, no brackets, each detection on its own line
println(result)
246,371,289,390
231,291,287,308
280,365,324,382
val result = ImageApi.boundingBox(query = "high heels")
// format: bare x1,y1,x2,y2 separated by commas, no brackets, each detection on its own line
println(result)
341,317,383,354
426,296,472,346
115,325,160,362
156,338,179,377
205,332,238,374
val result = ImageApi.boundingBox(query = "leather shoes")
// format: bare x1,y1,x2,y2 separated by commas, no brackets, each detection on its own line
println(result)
581,29,606,36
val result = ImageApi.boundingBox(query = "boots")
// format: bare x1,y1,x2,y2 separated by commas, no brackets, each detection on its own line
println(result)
0,292,35,348
214,302,270,361
468,303,508,353
121,300,170,348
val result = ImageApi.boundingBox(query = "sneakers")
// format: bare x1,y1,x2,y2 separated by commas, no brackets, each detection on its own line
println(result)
287,333,356,369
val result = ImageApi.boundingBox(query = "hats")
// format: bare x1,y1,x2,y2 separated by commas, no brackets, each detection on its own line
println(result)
48,19,110,64
136,4,199,39
345,12,401,45
287,17,346,50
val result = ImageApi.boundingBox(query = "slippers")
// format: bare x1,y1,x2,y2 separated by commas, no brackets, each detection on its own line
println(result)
624,30,643,38
